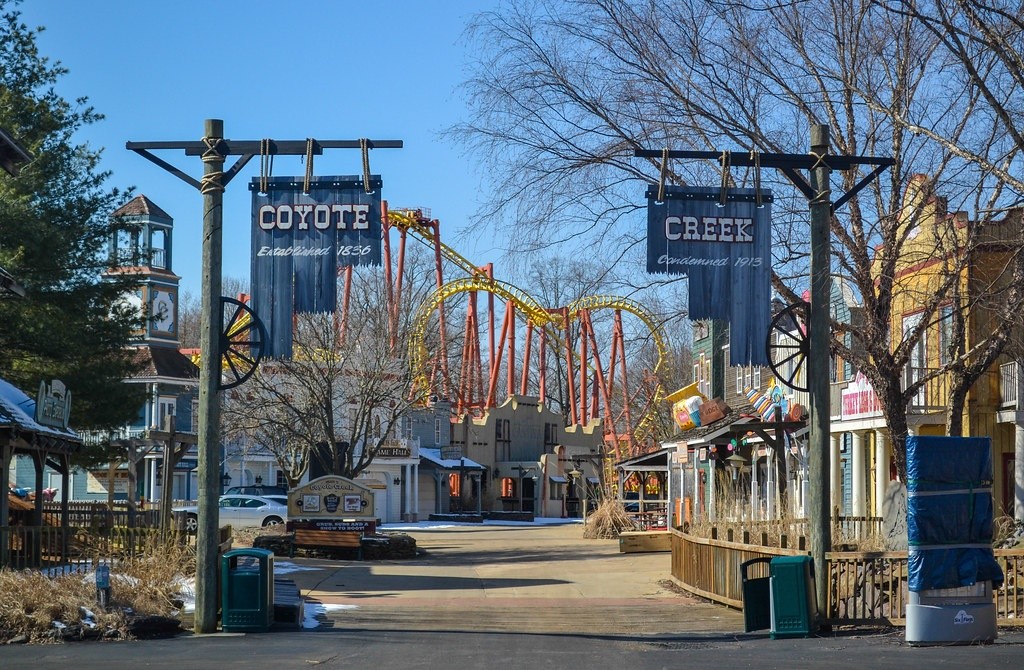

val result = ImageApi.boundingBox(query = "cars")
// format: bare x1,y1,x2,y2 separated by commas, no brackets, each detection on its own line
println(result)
172,494,287,534
217,485,286,507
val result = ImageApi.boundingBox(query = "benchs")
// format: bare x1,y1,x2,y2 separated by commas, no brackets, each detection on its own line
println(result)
290,528,363,562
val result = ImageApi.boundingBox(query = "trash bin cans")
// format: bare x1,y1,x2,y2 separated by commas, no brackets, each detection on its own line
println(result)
738,553,814,640
220,547,275,632
